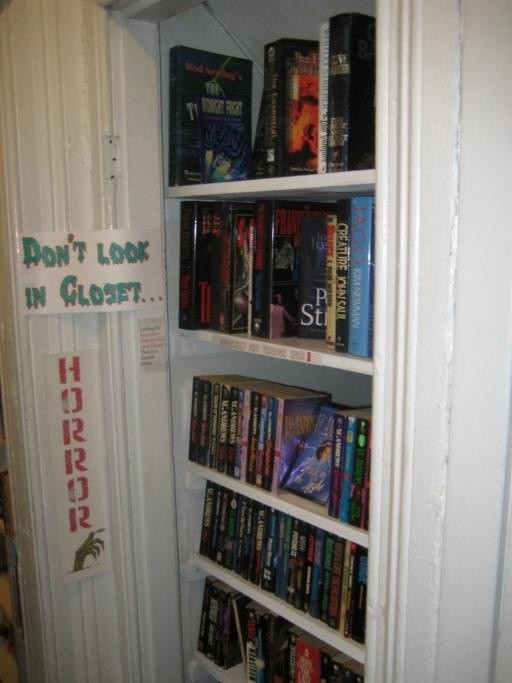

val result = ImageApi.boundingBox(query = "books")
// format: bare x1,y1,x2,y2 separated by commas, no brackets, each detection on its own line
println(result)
197,479,369,645
168,45,252,188
179,195,376,357
197,574,365,683
317,13,376,175
253,37,320,178
188,375,372,529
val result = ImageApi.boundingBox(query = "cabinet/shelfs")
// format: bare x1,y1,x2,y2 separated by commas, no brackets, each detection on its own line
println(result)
160,0,398,682
